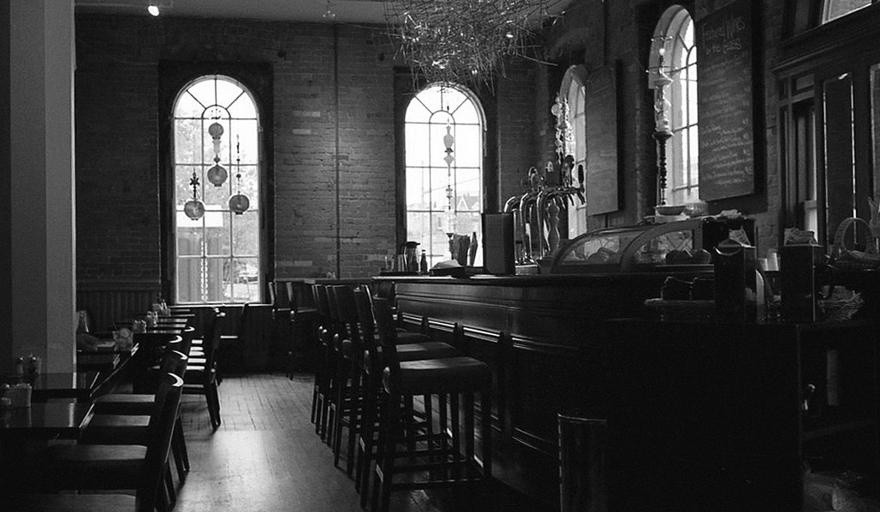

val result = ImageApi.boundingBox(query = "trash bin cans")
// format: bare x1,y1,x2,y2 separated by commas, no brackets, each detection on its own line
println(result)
556,413,608,512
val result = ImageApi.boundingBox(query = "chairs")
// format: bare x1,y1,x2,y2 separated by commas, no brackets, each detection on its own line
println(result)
269,278,493,511
24,301,251,512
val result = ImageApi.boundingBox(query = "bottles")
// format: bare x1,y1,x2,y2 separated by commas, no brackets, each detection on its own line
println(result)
420,249,428,272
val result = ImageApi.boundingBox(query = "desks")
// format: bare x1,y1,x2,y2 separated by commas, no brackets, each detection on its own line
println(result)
662,305,876,511
2,371,101,511
